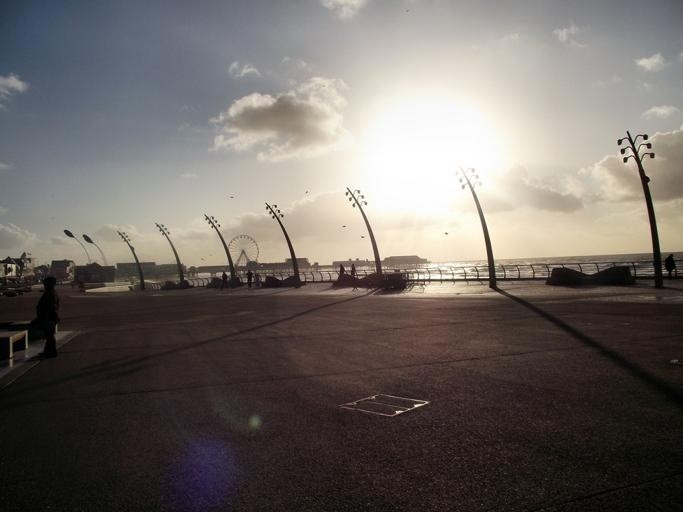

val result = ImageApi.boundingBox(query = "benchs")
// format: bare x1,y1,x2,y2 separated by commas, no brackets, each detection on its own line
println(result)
1,329,29,359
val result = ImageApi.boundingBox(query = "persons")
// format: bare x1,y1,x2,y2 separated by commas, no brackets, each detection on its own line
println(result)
35,276,61,359
222,271,228,288
339,263,357,276
246,269,253,287
664,253,676,280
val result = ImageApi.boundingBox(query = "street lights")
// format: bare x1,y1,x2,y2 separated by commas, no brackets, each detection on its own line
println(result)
63,230,90,262
265,203,301,280
618,131,664,288
117,231,146,289
205,214,237,277
456,167,496,288
83,234,109,265
346,187,383,273
155,223,185,281
